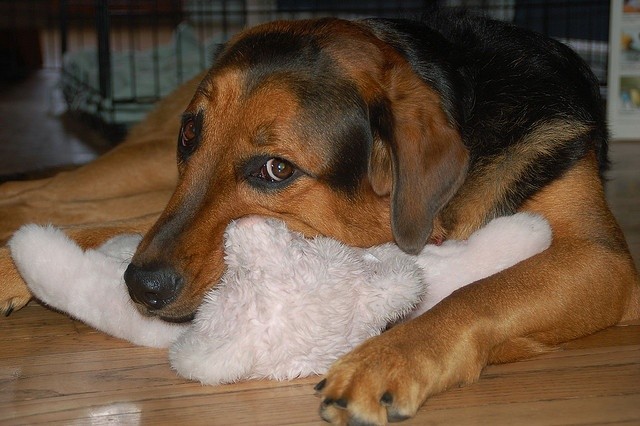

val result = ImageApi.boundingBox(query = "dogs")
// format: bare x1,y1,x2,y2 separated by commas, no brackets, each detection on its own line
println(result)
0,0,640,426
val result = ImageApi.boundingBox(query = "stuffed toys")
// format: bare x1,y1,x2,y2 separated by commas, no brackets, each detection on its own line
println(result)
11,210,552,385
